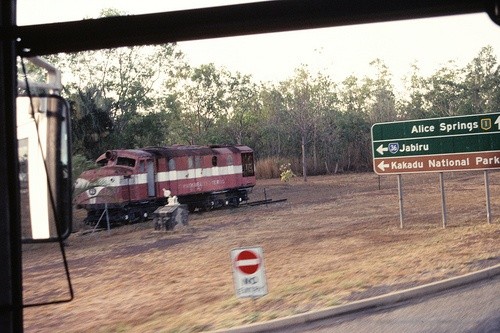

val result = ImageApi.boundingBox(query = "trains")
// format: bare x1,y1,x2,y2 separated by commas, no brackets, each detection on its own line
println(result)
72,144,255,229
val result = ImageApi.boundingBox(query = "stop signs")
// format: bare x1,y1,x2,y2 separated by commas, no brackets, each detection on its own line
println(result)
231,245,268,298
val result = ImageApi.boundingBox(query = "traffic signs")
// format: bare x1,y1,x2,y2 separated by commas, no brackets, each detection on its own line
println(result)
371,113,500,175
